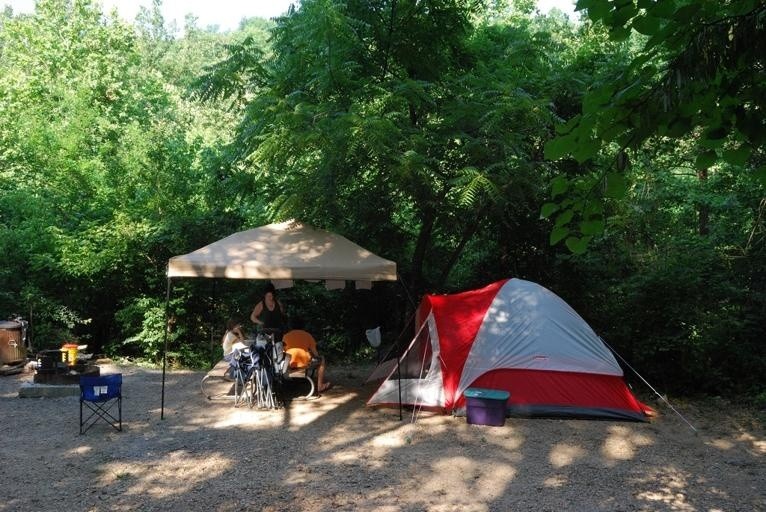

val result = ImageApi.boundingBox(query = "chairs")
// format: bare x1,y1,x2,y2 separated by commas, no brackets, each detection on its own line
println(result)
78,373,121,435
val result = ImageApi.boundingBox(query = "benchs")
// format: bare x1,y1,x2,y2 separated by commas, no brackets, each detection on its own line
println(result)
200,357,315,401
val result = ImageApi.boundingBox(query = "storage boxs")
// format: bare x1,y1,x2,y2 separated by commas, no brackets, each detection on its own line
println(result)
462,387,510,427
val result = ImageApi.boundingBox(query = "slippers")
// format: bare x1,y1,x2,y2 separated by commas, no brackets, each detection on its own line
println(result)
317,381,332,392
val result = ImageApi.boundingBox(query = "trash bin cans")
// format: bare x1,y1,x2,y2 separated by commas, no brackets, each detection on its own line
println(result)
0,318,29,365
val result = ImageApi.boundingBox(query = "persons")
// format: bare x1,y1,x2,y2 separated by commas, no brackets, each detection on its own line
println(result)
221,318,249,382
249,288,286,332
282,314,332,392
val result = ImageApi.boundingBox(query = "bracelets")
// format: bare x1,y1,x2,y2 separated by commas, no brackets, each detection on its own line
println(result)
238,335,244,338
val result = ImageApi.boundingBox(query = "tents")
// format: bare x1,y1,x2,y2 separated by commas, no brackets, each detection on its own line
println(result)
365,276,702,435
